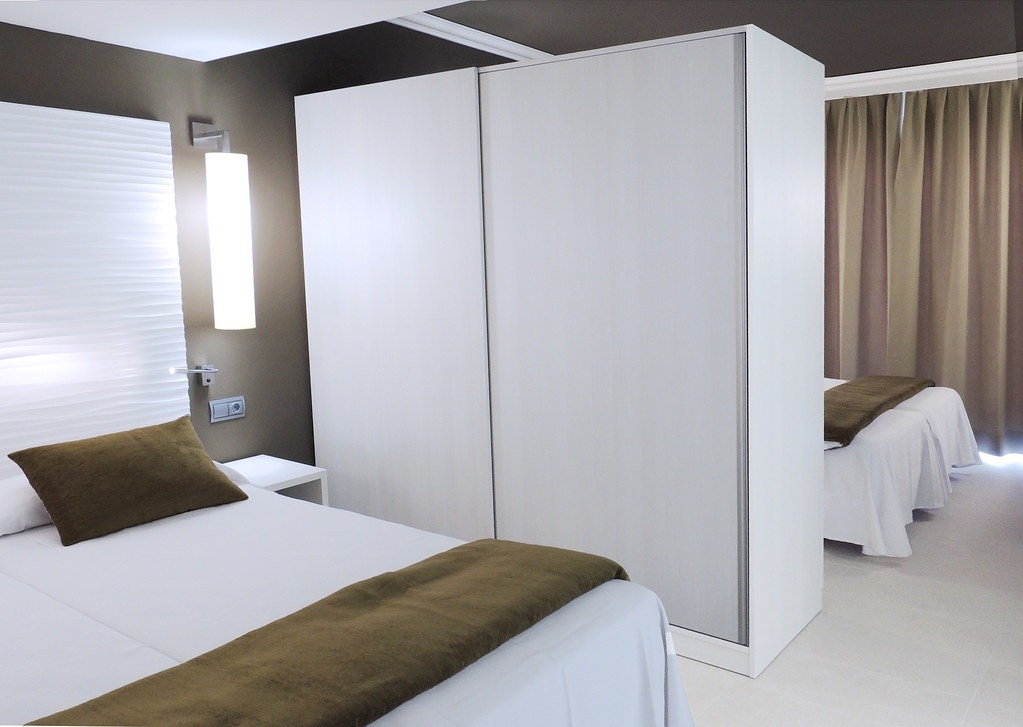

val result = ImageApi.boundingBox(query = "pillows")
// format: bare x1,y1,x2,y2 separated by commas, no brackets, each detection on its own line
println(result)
6,416,251,546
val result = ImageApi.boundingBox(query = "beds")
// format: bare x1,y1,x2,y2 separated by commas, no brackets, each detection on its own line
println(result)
0,457,691,727
824,374,980,559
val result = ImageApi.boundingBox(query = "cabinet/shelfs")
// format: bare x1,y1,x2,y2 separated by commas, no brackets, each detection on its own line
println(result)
293,21,825,680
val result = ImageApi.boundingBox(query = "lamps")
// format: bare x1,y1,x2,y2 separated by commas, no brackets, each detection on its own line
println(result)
168,363,219,387
191,122,258,332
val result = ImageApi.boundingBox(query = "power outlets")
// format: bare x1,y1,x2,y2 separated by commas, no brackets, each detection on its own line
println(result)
208,395,246,424
